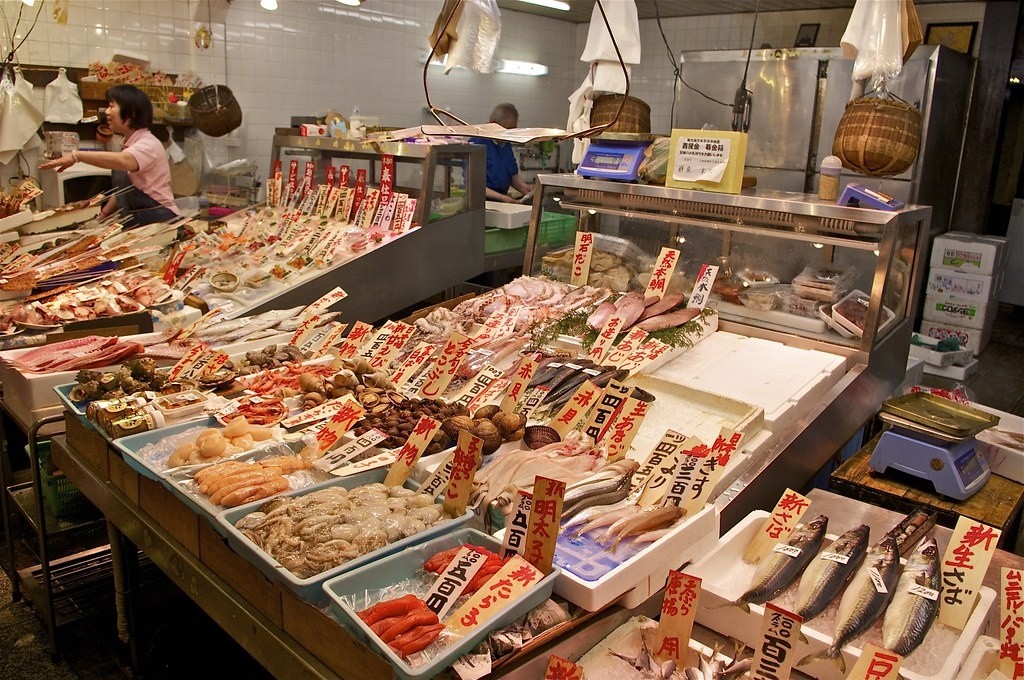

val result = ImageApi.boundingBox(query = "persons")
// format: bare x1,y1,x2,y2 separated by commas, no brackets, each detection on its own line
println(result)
461,102,533,204
38,84,179,230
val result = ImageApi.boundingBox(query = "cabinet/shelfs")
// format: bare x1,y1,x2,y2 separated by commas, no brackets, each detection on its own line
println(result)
0,414,153,666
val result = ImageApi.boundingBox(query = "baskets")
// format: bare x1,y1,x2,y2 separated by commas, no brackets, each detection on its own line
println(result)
188,84,242,137
138,86,170,124
591,93,651,132
832,96,922,176
164,95,194,125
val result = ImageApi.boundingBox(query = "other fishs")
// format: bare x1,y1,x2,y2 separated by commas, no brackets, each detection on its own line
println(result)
0,273,344,374
467,292,943,680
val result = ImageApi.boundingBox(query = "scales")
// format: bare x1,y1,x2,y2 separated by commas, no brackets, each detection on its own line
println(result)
866,390,1002,504
576,132,670,183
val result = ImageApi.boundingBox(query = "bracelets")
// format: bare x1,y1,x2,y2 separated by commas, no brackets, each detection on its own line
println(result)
71,150,79,163
102,210,108,216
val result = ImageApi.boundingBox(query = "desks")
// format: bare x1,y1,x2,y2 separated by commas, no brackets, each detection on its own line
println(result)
830,429,1024,550
50,434,337,680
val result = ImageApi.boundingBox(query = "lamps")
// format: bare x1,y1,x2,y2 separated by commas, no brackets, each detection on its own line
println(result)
195,0,212,50
419,46,549,78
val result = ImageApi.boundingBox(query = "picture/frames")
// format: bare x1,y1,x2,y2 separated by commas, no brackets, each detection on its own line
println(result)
923,21,979,54
793,23,821,47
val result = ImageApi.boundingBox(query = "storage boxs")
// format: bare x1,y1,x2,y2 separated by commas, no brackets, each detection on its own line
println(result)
681,509,1000,680
902,385,1024,486
80,81,199,121
0,205,895,680
202,173,255,207
664,128,748,194
908,332,978,380
921,230,1011,356
484,199,576,254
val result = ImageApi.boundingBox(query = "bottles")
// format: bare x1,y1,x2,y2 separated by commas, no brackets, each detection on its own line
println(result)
818,155,842,201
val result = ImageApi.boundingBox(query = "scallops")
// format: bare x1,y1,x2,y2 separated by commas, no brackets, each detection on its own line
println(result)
473,421,502,456
439,416,474,444
492,412,507,427
474,418,495,429
474,405,502,420
497,412,527,442
524,425,561,450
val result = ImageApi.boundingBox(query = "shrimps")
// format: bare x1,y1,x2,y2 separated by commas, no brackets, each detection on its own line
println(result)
220,359,340,424
